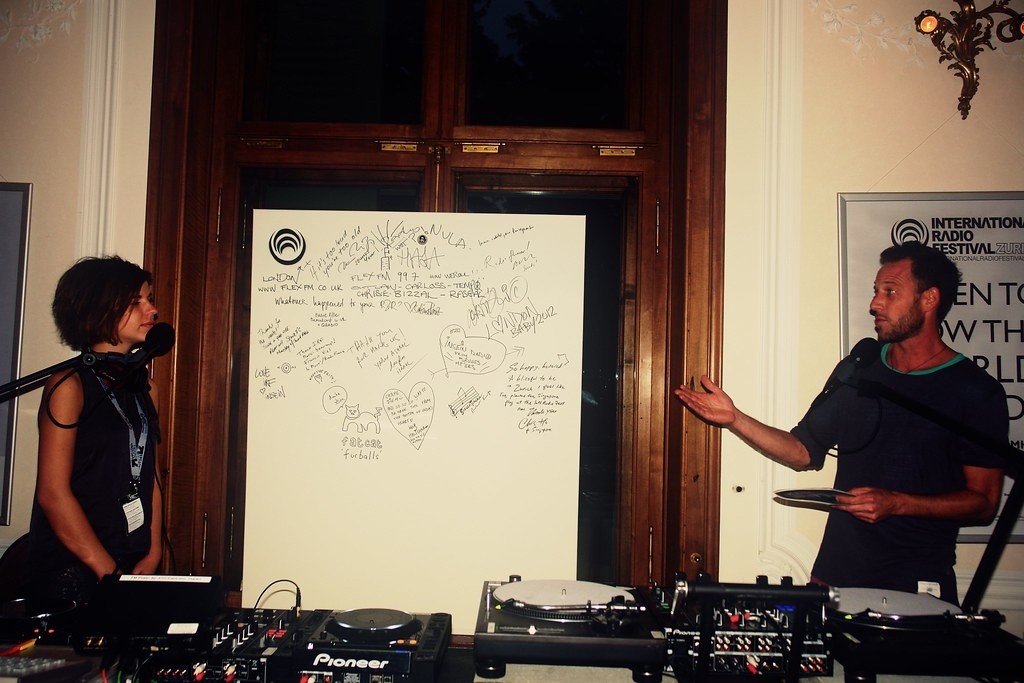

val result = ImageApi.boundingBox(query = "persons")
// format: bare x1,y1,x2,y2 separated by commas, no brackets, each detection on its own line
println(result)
30,254,166,599
673,240,1011,605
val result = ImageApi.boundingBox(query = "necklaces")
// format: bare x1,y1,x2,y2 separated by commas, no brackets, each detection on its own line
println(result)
888,342,948,374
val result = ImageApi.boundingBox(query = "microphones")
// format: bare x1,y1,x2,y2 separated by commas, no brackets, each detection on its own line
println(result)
106,323,175,395
